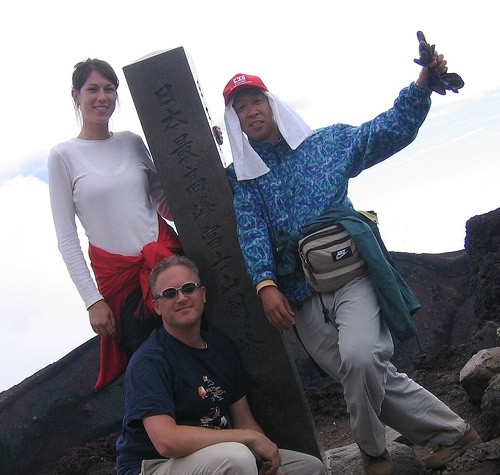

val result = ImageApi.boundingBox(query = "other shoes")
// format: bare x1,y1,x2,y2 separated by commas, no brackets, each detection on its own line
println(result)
425,426,482,469
359,446,393,475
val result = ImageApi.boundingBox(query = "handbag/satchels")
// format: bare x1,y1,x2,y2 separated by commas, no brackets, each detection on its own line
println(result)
296,210,380,294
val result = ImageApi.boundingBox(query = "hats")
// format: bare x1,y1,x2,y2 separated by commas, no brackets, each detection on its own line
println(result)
222,73,269,107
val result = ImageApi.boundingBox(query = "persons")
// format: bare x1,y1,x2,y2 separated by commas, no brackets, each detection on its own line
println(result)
222,50,481,475
46,58,185,391
116,255,327,475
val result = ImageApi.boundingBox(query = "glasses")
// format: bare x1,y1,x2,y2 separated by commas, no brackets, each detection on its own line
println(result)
154,282,202,301
